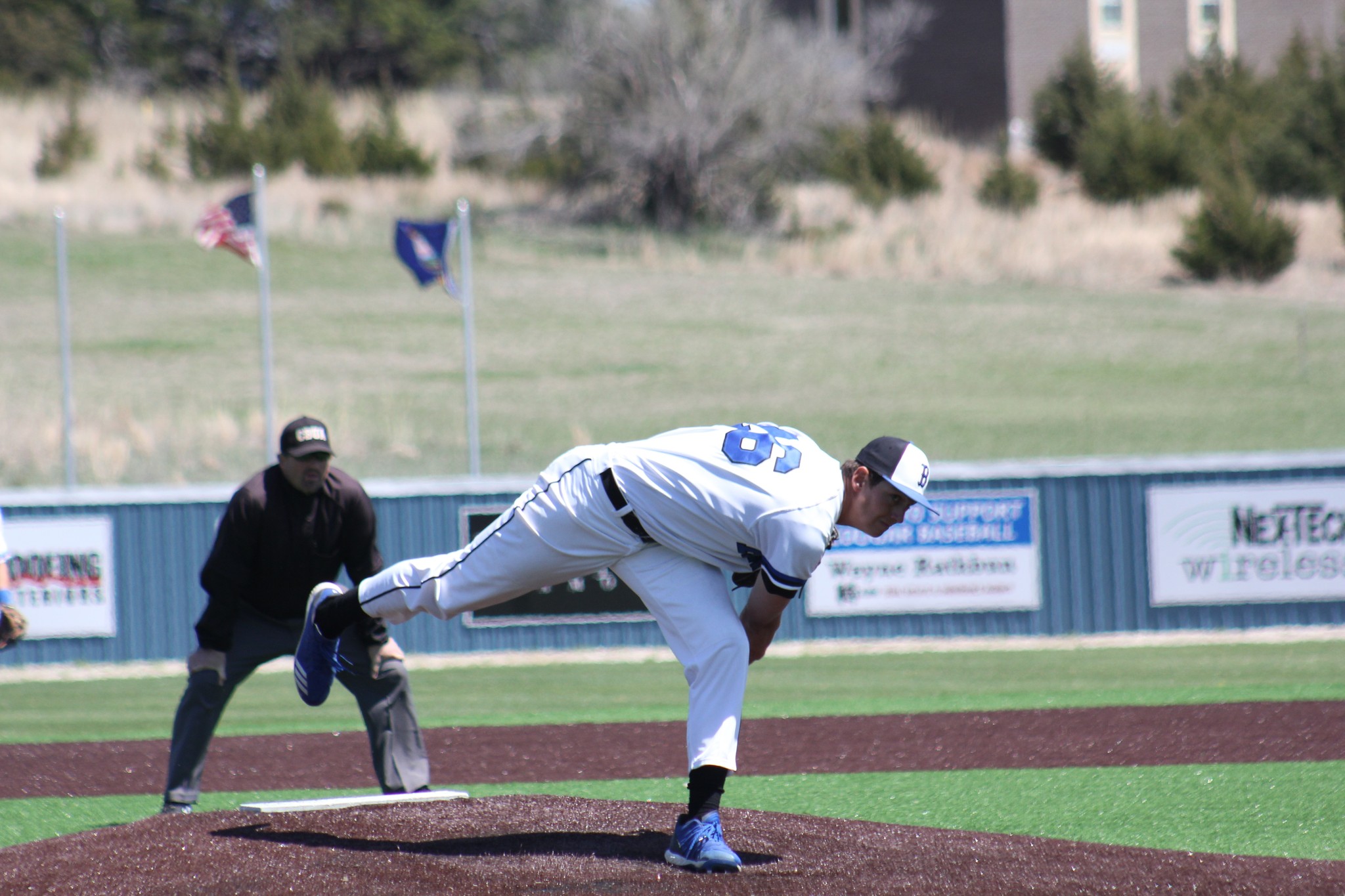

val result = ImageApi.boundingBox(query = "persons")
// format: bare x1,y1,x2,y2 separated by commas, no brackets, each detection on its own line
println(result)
294,421,942,872
162,414,433,815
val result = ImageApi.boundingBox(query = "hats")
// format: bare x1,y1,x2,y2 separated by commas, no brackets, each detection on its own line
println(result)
855,436,939,520
280,416,336,459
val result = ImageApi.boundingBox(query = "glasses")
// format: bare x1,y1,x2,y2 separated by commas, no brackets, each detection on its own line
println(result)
289,452,331,461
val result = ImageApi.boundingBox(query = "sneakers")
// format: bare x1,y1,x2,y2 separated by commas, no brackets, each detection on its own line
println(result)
664,810,743,873
294,582,353,706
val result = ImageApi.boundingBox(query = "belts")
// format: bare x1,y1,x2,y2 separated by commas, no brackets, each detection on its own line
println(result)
600,468,657,543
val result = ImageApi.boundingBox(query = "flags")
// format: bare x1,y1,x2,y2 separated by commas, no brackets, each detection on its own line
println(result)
396,217,454,290
194,194,263,270
